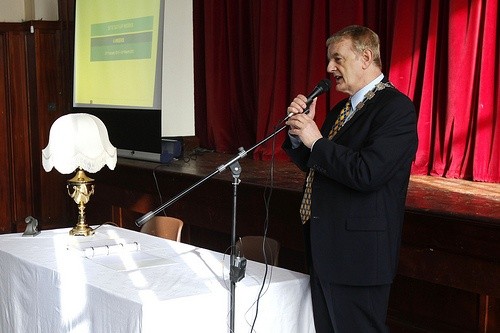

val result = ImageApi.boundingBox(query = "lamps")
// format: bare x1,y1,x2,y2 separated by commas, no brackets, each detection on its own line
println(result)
41,113,117,236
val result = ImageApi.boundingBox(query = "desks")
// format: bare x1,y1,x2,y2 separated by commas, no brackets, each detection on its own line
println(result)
59,150,500,333
0,225,316,333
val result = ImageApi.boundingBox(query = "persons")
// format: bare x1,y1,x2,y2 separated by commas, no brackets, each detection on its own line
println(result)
285,25,418,333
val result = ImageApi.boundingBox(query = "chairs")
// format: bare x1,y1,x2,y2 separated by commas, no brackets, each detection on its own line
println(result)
140,216,183,242
236,236,279,266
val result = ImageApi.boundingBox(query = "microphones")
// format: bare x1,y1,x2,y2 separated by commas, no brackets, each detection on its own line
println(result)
284,80,329,120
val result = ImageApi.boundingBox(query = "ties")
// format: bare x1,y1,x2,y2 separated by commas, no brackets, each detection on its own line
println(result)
299,102,352,224
